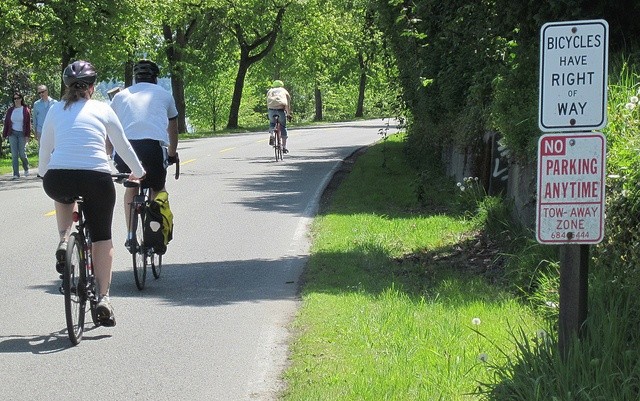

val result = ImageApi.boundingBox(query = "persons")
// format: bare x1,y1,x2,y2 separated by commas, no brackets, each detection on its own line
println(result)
2,92,31,179
266,79,291,154
35,60,147,327
105,60,179,253
33,85,58,177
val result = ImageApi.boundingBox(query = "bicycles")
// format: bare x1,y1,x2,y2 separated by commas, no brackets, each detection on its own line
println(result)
110,152,180,290
267,114,293,162
36,172,147,344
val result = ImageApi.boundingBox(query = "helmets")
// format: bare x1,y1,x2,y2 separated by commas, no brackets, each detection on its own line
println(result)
133,58,160,82
62,59,99,88
273,79,284,88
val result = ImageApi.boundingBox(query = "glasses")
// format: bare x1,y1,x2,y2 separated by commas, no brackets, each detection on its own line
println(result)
37,89,48,94
13,96,22,99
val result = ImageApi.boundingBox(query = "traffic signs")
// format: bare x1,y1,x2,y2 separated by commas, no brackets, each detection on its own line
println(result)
538,19,609,132
535,132,605,245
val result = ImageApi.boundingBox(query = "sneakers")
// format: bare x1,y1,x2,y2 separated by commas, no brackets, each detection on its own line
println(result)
96,298,117,327
24,169,29,176
283,147,289,152
125,238,140,254
13,174,20,179
269,136,274,144
54,239,68,274
